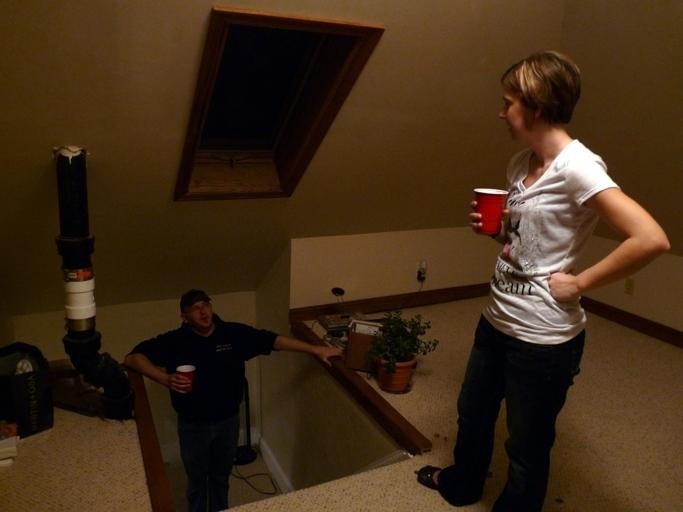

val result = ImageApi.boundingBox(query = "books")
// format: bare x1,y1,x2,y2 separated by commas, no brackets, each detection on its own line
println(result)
319,312,363,339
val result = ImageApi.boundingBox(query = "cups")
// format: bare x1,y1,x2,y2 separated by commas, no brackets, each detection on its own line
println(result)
473,187,510,237
175,364,197,393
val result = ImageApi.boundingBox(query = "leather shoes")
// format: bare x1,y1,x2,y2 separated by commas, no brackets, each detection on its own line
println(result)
418,466,442,490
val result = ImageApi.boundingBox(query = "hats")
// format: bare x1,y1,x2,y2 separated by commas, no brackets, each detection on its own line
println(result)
181,290,213,311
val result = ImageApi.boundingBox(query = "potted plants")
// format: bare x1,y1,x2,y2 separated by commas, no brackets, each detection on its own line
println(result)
372,313,435,397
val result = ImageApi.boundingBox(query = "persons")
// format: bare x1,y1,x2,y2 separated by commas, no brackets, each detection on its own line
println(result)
411,49,672,511
123,287,345,511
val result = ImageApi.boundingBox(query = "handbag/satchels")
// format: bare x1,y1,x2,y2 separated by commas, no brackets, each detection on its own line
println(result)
233,445,257,465
0,342,53,438
343,319,412,372
